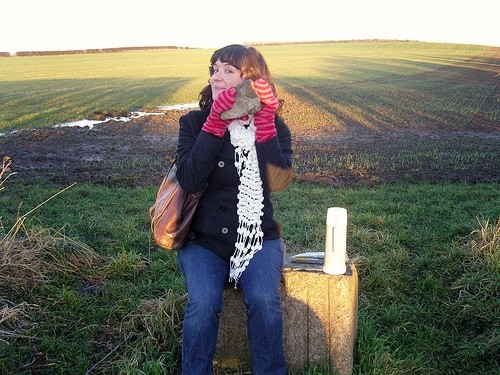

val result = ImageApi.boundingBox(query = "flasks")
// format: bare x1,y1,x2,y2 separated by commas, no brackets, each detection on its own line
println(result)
322,207,348,274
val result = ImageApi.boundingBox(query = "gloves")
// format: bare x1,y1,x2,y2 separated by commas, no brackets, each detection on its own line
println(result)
202,86,249,139
253,77,279,144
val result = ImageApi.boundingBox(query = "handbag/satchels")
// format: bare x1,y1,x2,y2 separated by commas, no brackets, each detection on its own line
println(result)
148,154,209,251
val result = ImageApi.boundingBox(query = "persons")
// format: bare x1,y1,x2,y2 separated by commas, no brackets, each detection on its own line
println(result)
169,43,297,375
